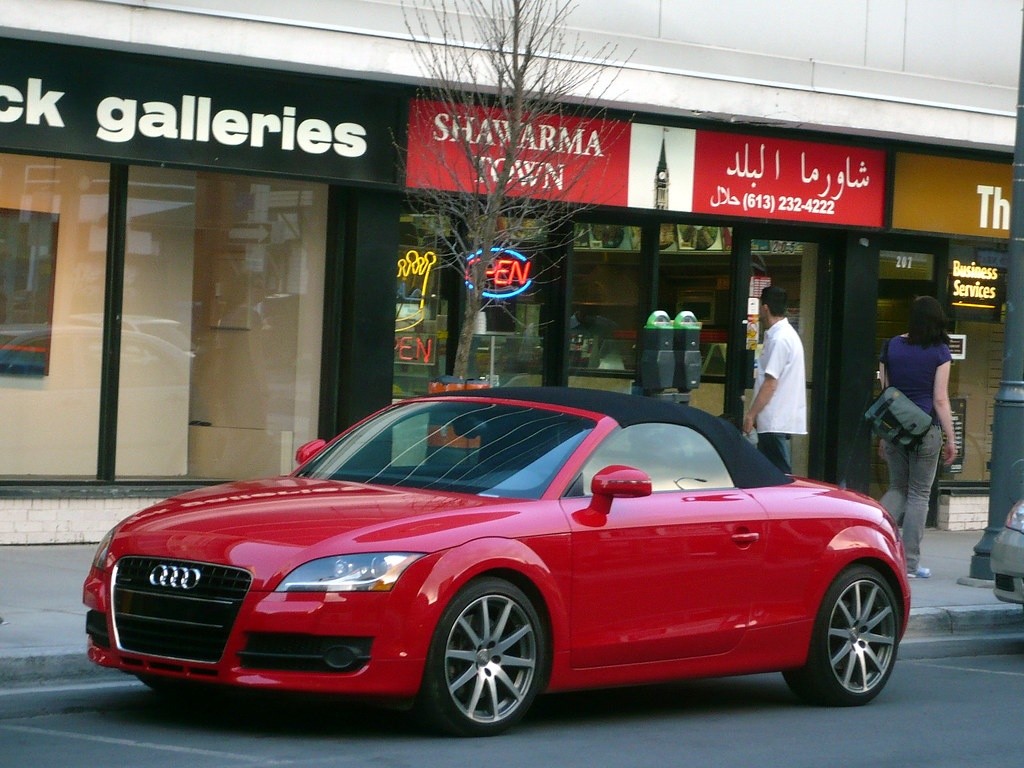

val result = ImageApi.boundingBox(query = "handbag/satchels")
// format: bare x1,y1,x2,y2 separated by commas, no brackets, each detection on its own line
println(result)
864,386,932,457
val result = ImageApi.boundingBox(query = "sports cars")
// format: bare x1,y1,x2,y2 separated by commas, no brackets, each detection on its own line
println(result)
79,384,914,737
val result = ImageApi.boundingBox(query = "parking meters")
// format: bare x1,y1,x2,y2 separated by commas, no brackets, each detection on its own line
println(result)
677,310,704,404
641,307,675,403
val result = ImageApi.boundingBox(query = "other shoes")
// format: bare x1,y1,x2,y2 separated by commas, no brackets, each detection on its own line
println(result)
907,564,931,578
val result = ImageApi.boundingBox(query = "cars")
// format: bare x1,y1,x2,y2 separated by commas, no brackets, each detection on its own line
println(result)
990,504,1024,605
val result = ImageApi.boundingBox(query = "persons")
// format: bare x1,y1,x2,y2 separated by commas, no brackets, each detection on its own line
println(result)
743,286,808,476
879,296,956,579
